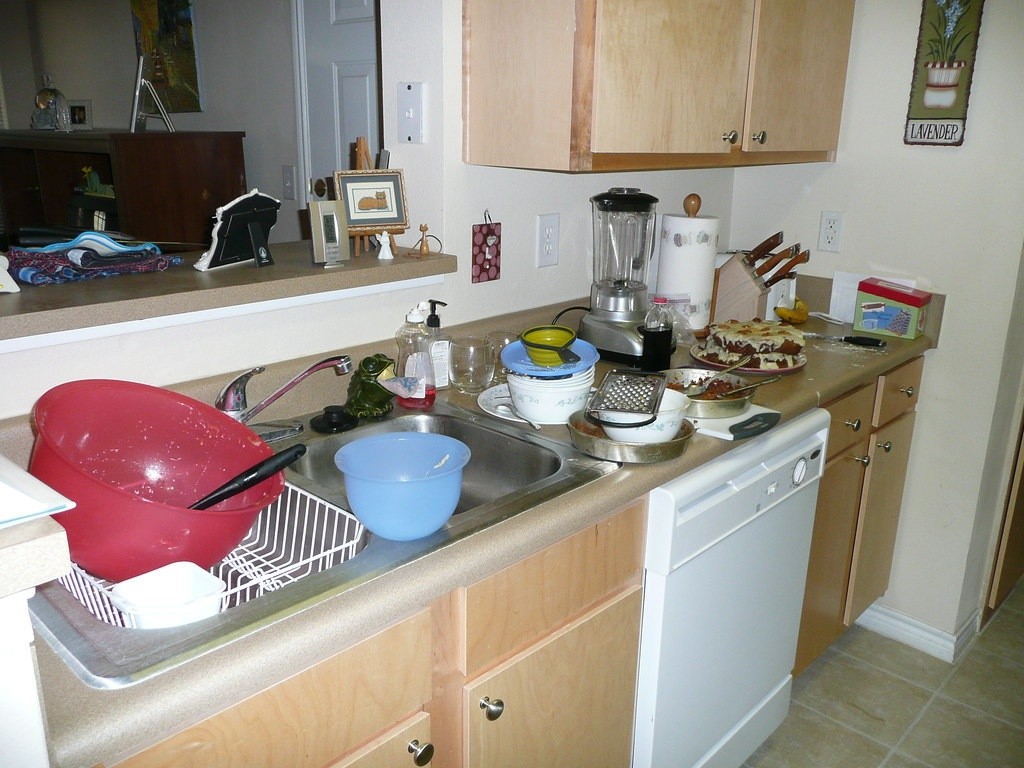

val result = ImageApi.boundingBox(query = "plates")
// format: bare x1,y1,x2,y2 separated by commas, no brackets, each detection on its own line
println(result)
477,383,598,425
689,341,807,373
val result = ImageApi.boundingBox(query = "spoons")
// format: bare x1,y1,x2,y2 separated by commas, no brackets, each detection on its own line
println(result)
681,356,755,396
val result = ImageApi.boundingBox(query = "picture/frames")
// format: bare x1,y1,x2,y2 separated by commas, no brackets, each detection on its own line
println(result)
334,168,411,232
65,99,93,130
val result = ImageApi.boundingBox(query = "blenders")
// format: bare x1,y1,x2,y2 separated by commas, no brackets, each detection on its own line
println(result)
578,187,677,367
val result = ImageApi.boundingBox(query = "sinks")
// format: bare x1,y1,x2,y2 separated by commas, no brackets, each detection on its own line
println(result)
27,464,376,693
276,408,624,579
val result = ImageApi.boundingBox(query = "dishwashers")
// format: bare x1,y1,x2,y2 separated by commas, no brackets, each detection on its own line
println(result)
630,406,831,768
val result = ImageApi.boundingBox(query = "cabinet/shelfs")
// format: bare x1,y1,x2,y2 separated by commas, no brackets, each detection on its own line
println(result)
0,127,246,254
114,493,650,768
463,0,855,172
793,356,925,679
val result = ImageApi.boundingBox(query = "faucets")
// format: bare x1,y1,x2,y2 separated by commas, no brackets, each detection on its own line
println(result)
215,354,353,425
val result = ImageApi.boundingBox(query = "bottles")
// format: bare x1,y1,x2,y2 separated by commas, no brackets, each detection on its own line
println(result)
644,296,673,331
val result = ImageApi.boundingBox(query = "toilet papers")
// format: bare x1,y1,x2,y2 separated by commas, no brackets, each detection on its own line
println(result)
655,214,720,329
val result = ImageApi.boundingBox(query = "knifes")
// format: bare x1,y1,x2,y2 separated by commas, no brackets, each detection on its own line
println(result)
742,231,810,288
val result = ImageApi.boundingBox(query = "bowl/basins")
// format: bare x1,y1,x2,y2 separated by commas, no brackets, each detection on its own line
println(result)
656,369,757,419
506,363,596,419
334,432,472,542
521,325,581,367
27,379,285,583
111,561,227,627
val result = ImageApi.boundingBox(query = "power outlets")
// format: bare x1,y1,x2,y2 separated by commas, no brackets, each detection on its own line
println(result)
818,209,843,255
535,213,560,268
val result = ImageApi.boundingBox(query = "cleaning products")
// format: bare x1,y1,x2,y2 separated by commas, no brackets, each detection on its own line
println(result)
426,299,453,391
394,301,437,412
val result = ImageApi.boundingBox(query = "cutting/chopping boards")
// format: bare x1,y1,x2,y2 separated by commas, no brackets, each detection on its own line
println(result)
684,404,782,441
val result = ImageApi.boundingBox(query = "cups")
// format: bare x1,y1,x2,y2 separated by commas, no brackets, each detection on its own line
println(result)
448,337,495,393
484,331,521,381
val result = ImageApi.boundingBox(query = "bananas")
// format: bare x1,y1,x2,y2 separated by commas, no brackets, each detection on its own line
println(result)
773,296,808,323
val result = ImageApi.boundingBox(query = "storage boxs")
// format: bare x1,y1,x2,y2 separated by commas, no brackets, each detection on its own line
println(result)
853,276,933,339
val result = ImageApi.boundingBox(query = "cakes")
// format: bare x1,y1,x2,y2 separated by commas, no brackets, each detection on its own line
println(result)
698,316,805,369
